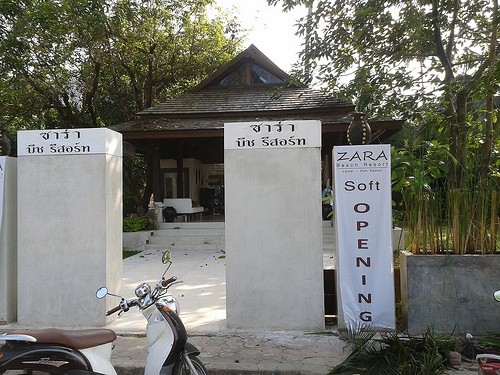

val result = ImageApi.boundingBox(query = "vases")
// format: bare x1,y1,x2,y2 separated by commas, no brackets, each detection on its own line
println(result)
164,207,176,223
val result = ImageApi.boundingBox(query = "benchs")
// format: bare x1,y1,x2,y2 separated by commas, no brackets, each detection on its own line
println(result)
164,198,204,222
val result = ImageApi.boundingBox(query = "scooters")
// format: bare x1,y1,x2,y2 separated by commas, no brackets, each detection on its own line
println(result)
474,290,500,375
0,248,208,375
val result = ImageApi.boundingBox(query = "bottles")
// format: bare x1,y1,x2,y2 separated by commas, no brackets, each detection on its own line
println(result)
162,206,177,222
